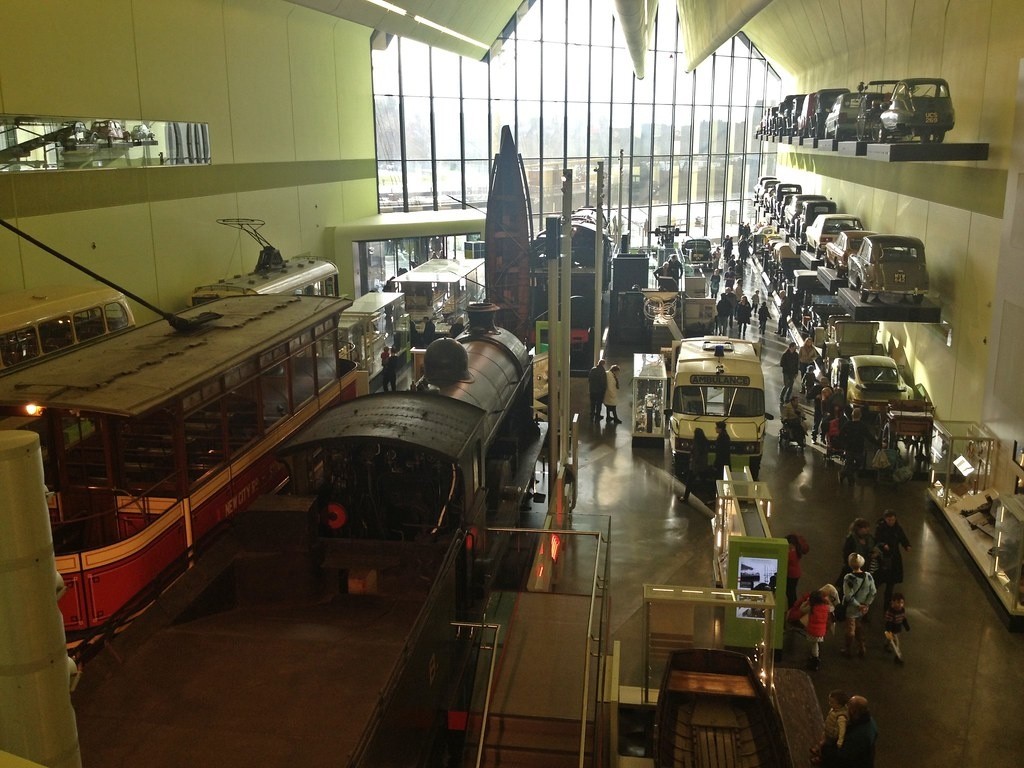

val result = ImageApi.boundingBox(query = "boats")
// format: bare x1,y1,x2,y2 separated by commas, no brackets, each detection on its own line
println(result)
338,257,483,382
0,216,359,630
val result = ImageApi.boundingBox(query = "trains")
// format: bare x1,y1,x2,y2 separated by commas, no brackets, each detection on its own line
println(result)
73,203,616,767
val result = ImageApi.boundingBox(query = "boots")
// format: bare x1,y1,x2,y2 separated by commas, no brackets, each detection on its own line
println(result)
805,657,820,671
841,635,866,657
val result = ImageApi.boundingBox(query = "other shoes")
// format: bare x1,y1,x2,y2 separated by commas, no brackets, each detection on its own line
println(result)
894,656,904,666
606,417,622,423
884,644,894,654
809,744,822,754
591,413,604,419
810,756,822,765
678,496,688,504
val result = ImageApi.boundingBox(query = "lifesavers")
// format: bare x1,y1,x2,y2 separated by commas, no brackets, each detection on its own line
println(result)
466,529,482,552
326,504,346,527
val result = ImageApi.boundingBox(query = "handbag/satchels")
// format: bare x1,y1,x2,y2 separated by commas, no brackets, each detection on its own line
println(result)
834,603,846,622
870,449,893,468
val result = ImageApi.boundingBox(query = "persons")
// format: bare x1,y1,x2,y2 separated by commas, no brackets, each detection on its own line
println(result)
784,509,911,671
710,222,750,298
717,277,772,335
588,359,623,423
653,254,683,285
422,316,435,346
779,336,887,487
380,346,401,391
678,421,732,504
450,318,464,339
810,690,877,768
774,290,791,337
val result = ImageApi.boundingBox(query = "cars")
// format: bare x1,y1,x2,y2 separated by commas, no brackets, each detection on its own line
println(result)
756,78,956,143
67,120,157,145
755,175,931,304
749,226,917,417
683,236,714,266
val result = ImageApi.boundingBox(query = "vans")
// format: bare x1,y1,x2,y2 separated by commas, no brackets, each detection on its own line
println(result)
671,335,773,475
